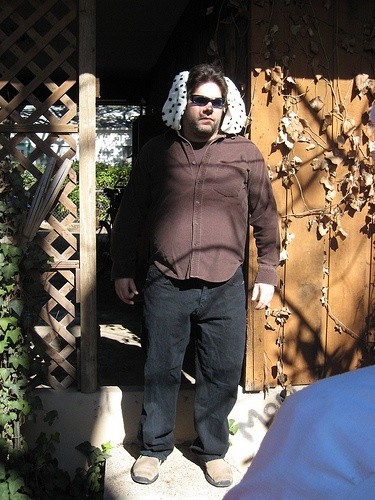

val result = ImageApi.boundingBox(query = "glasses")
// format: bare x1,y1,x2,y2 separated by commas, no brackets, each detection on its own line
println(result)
187,96,227,108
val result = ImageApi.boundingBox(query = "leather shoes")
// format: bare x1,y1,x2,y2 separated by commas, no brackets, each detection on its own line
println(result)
131,454,164,484
204,459,234,488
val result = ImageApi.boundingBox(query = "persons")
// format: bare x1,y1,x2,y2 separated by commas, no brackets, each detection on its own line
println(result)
223,363,374,500
109,64,281,488
